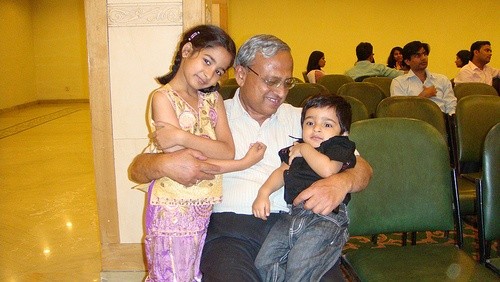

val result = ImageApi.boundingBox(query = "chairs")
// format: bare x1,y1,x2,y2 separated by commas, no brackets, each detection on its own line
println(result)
219,70,500,282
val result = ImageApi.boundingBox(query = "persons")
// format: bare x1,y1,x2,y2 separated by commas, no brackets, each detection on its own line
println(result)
344,42,409,82
128,34,373,282
387,47,410,71
453,41,500,87
144,24,268,282
450,50,471,87
389,40,457,116
252,93,356,282
307,51,326,83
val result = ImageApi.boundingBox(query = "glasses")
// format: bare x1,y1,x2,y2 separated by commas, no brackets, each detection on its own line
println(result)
247,66,296,90
415,51,427,57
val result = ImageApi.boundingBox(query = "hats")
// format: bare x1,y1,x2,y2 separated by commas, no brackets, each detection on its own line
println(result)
457,50,472,65
